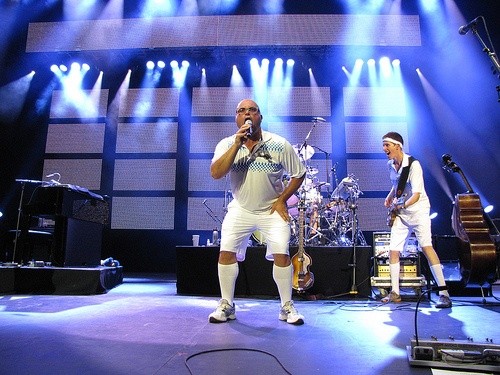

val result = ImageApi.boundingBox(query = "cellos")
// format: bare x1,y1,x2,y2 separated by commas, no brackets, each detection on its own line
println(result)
442,154,500,299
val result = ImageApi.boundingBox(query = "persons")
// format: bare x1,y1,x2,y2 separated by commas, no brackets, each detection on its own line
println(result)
380,132,452,309
209,99,308,325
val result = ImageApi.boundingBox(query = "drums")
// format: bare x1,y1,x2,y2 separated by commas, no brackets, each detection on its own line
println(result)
297,178,323,209
252,229,267,245
322,200,350,221
303,204,320,234
286,194,300,220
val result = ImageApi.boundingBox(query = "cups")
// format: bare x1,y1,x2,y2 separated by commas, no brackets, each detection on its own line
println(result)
193,235,199,246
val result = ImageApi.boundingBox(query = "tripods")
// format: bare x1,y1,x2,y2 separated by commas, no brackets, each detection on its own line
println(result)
289,120,376,300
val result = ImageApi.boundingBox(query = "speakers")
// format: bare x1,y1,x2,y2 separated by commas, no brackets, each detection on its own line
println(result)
428,258,492,297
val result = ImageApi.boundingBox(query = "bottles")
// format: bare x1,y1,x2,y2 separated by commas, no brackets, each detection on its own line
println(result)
212,227,218,246
207,238,210,246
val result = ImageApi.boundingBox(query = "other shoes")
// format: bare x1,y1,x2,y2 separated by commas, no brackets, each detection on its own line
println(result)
381,290,402,303
435,295,452,308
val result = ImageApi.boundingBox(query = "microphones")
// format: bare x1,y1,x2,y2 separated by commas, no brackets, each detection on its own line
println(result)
313,117,326,122
458,16,482,36
245,119,253,135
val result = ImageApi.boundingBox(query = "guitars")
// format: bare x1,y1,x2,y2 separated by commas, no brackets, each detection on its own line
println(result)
387,194,405,227
291,201,314,292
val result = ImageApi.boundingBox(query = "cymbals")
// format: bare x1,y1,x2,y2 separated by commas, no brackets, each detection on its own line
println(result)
306,168,319,175
293,144,315,161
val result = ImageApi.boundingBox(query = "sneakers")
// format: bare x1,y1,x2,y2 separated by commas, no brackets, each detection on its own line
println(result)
207,299,237,323
278,300,305,325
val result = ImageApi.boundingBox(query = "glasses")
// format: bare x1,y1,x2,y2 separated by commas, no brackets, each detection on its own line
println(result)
235,106,260,115
